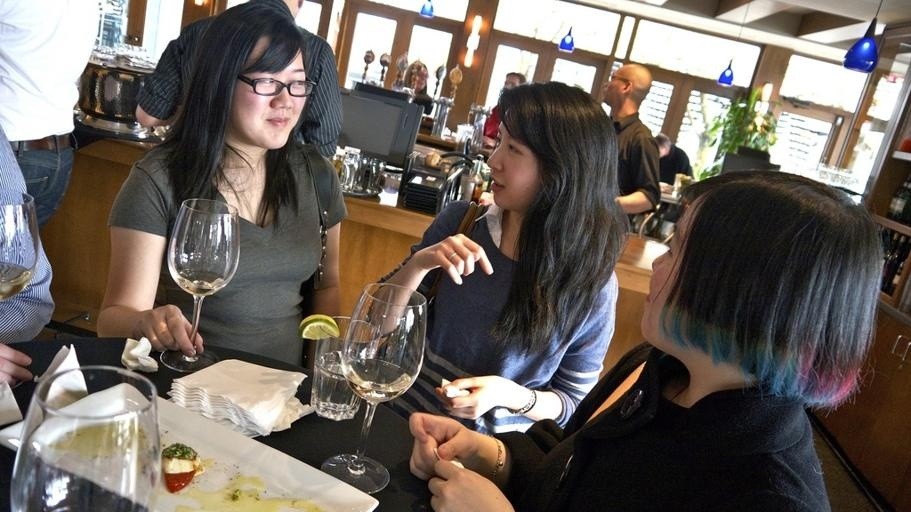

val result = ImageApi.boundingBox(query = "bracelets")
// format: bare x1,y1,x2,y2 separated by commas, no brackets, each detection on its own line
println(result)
491,434,504,483
507,389,537,416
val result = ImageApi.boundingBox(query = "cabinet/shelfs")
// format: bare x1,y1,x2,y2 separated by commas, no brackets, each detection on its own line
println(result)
807,68,910,509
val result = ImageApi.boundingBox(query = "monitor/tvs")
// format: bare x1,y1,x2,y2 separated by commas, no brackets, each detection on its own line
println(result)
338,87,425,168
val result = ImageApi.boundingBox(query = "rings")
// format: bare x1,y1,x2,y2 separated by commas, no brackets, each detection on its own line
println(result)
449,252,457,259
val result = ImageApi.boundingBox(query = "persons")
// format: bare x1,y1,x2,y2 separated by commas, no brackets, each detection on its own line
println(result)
97,8,348,369
0,0,101,233
367,80,629,435
135,1,344,159
398,61,434,115
600,64,661,220
482,72,527,151
0,124,56,389
654,133,694,192
410,168,885,512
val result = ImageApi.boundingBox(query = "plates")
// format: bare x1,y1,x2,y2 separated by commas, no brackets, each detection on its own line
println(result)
0,393,379,512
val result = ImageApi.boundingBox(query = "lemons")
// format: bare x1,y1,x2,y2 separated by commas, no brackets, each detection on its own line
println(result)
299,313,340,341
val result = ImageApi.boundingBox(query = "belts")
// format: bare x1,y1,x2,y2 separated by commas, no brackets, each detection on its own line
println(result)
6,133,70,151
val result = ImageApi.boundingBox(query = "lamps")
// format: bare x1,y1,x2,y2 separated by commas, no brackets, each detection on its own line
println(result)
718,0,751,89
840,1,884,75
556,0,582,55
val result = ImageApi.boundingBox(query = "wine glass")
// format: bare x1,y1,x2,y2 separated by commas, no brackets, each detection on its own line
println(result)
0,186,39,302
160,198,240,373
9,364,162,512
320,283,429,495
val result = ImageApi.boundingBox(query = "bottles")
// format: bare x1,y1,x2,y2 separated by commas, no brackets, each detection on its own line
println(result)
886,177,911,223
673,172,684,199
879,225,911,297
469,154,484,203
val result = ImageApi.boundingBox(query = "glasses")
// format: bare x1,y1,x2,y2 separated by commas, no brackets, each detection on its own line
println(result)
237,73,316,97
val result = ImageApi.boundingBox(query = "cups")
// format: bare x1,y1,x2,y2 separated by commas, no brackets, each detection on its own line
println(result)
455,123,474,144
330,150,388,193
310,316,381,421
114,42,150,57
460,175,476,203
